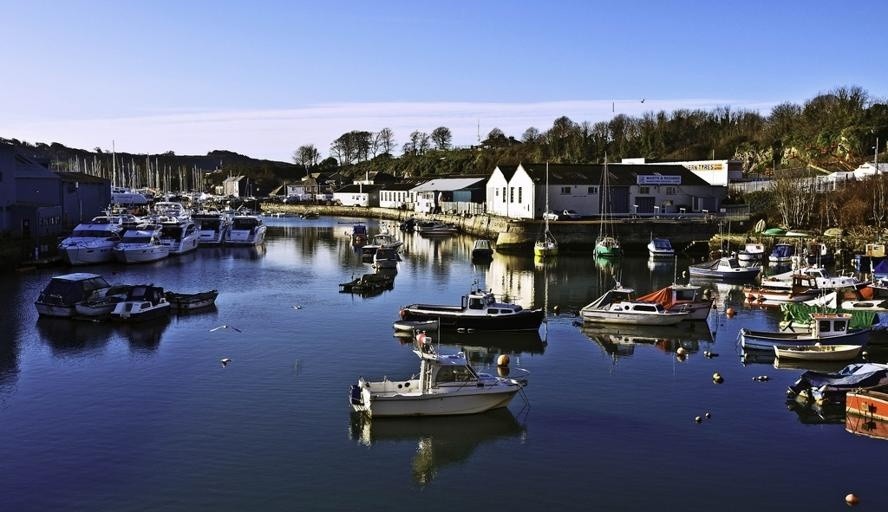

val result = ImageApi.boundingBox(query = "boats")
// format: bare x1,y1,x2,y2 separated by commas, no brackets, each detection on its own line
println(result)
580,265,689,327
56,201,268,265
739,208,888,324
785,362,888,418
772,342,863,361
582,320,692,377
399,280,545,330
647,231,675,257
772,357,864,374
393,319,439,332
681,218,761,285
401,216,457,237
633,253,716,322
471,227,494,258
740,346,776,367
338,221,404,293
845,383,888,421
35,272,219,323
736,311,881,353
345,329,532,419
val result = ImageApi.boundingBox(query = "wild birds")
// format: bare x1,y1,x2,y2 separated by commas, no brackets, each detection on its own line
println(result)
211,325,242,334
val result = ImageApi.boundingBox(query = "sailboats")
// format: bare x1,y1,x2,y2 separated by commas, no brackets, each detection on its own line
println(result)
593,150,625,258
533,160,561,259
